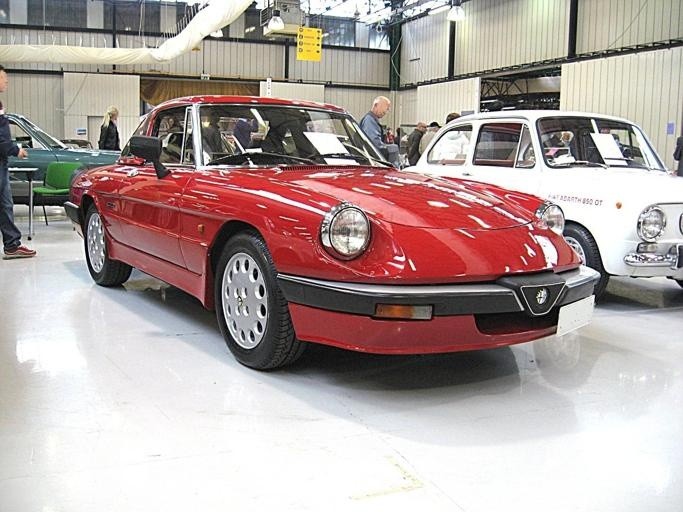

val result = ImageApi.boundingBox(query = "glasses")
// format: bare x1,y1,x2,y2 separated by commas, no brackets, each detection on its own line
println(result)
419,126,427,129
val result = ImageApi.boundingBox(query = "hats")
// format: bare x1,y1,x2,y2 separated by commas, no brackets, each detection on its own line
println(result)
429,121,442,129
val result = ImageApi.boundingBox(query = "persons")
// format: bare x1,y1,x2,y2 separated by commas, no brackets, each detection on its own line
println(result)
208,112,223,156
357,95,401,167
672,135,683,178
404,122,428,165
393,129,406,144
162,117,185,134
418,121,442,156
0,65,38,259
96,104,122,149
435,112,468,156
233,119,260,155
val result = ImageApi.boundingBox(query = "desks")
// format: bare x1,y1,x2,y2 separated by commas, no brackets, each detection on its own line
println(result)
7,167,40,240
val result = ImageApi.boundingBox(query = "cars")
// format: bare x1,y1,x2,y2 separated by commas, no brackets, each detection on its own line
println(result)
402,109,683,303
1,112,124,207
63,95,603,371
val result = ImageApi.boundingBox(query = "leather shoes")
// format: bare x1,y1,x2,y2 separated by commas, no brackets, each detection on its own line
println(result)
3,245,38,258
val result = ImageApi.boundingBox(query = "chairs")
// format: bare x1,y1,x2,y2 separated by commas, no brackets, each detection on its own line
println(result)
184,125,222,163
32,161,86,233
568,125,595,164
